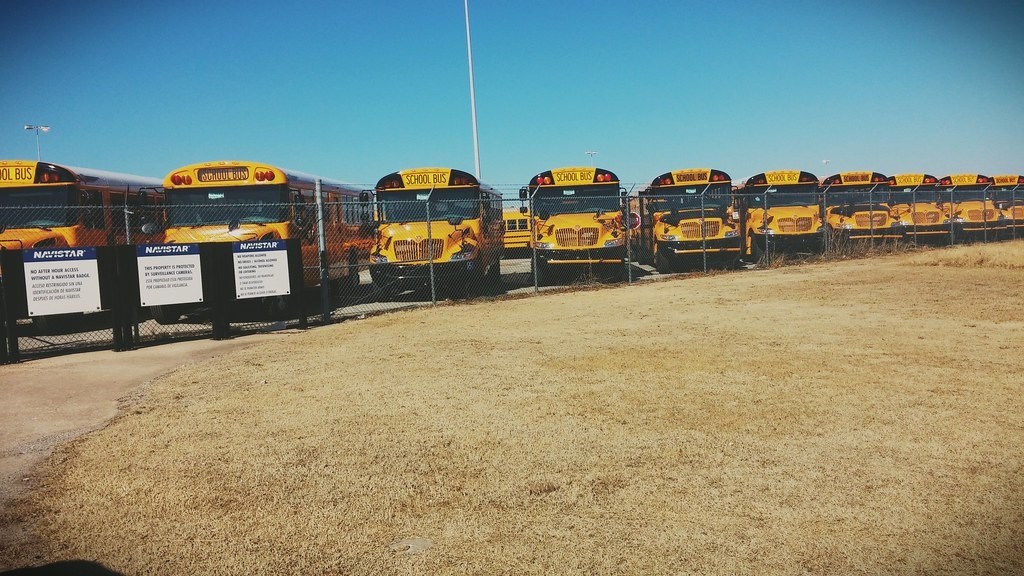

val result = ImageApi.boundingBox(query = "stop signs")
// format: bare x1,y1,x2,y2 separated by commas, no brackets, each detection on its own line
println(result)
626,212,642,231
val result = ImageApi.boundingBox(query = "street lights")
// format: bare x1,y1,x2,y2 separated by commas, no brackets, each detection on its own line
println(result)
24,124,52,161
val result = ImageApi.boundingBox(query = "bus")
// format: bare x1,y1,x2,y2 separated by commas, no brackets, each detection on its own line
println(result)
134,159,375,325
358,166,506,301
518,165,629,287
621,167,740,276
500,206,532,260
0,159,164,333
731,169,1024,264
